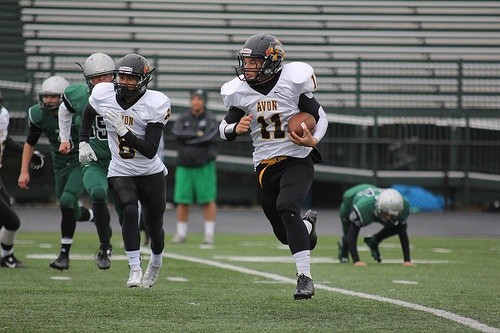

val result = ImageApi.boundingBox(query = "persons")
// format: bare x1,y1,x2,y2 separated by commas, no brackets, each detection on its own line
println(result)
338,184,414,266
79,54,171,287
58,53,141,269
219,34,328,298
0,107,44,268
172,89,219,249
19,76,94,270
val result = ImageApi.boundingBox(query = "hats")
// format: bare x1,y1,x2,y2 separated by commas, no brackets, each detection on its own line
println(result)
190,89,207,100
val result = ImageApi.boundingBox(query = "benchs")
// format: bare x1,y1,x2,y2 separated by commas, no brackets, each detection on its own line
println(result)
18,0,500,124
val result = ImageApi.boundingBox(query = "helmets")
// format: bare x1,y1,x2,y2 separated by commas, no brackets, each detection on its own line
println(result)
36,76,71,117
74,52,116,79
234,33,285,86
112,53,156,103
373,187,404,225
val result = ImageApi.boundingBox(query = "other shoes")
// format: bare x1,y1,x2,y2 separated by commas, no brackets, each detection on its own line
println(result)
49,255,70,271
364,235,381,263
1,253,24,268
338,235,349,263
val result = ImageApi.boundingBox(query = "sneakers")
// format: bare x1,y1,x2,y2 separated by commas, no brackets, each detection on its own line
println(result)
94,243,112,269
126,266,143,287
293,272,315,299
302,210,318,251
141,258,162,287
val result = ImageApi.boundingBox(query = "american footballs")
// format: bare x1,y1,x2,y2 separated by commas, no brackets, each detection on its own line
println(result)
287,112,316,139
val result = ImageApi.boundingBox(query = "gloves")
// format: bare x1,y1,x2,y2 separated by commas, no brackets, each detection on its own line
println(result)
77,141,97,165
105,109,129,137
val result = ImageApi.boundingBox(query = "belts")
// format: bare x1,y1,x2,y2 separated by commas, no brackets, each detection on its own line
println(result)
258,156,287,188
